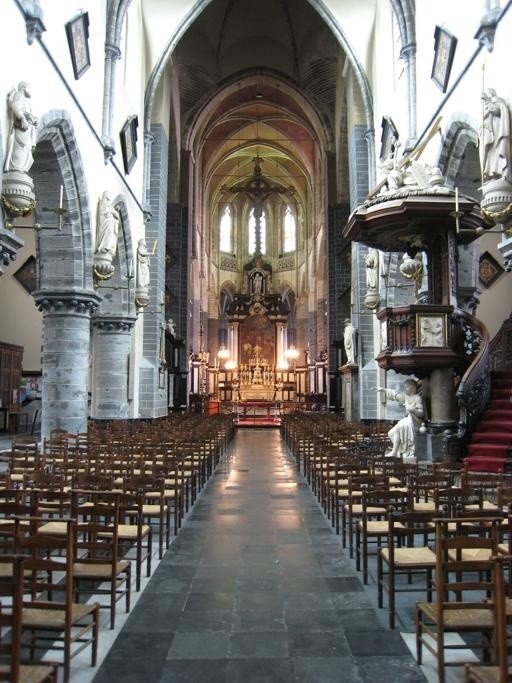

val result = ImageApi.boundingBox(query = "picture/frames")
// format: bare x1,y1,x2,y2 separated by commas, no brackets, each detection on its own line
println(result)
479,250,505,289
430,24,458,93
378,115,399,163
120,114,139,176
12,254,36,294
65,11,91,81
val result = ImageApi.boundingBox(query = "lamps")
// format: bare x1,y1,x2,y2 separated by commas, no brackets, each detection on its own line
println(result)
276,176,299,369
216,175,237,371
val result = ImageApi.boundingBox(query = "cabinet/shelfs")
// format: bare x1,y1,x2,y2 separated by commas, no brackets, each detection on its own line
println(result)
0,341,24,432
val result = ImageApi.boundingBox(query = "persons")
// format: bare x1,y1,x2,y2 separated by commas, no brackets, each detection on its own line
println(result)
343,319,356,364
364,247,379,292
4,82,38,175
167,319,174,336
96,191,119,255
371,379,425,459
253,273,262,293
479,88,511,183
137,239,150,288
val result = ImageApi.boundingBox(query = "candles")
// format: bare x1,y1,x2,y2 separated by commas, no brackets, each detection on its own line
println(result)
59,184,63,209
455,187,459,212
